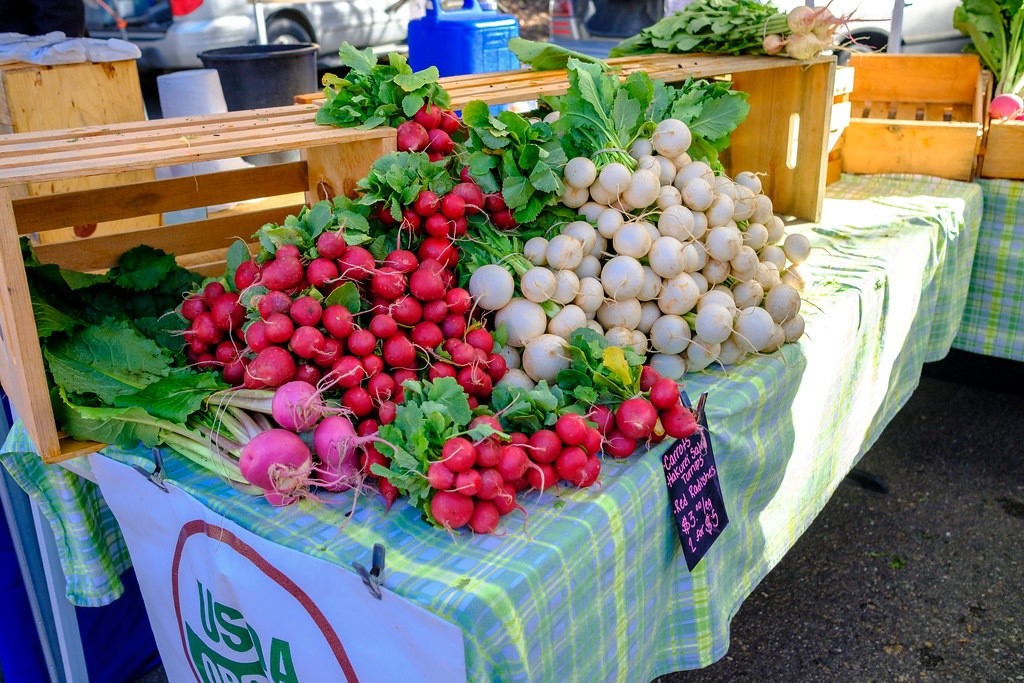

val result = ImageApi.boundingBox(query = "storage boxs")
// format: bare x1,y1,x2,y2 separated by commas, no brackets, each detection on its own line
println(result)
0,105,398,465
602,54,838,223
843,52,984,181
292,55,626,222
979,70,1024,181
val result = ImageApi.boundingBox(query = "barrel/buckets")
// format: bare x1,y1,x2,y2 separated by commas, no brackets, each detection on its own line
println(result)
196,41,320,108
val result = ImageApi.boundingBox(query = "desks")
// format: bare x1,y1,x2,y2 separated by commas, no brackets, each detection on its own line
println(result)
0,174,986,683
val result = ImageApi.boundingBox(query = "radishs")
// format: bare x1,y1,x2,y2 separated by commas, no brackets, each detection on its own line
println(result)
470,120,811,394
